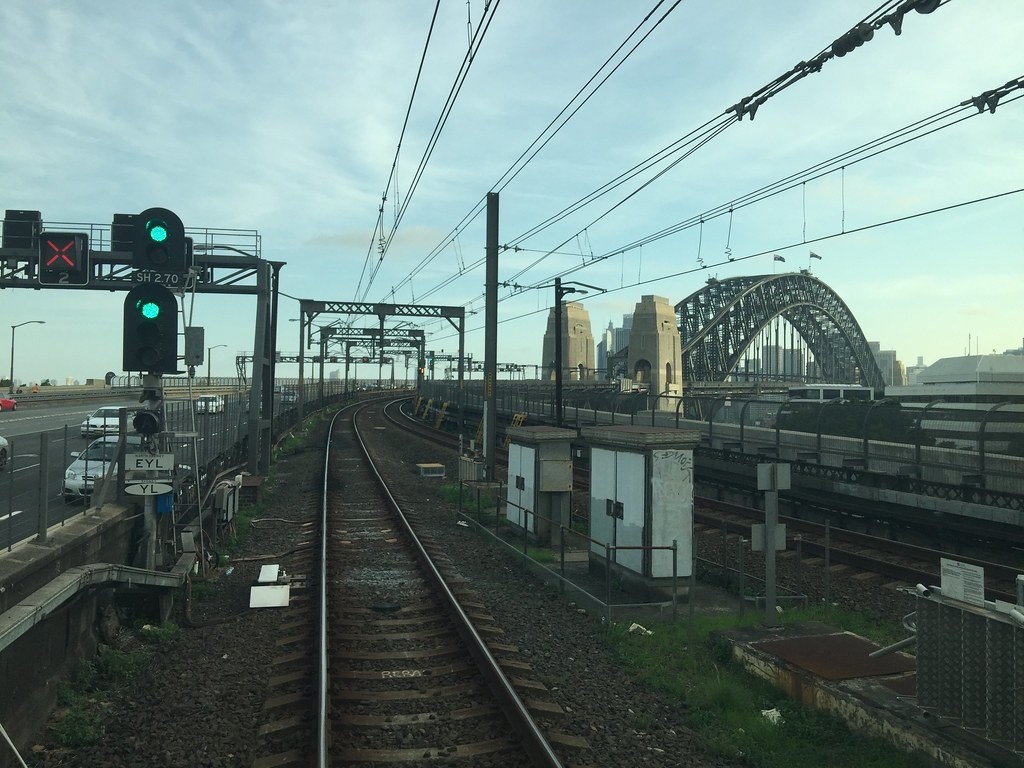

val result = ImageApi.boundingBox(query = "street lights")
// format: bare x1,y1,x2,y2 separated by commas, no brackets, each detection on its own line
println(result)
556,290,587,427
8,321,45,394
208,345,227,386
288,318,324,410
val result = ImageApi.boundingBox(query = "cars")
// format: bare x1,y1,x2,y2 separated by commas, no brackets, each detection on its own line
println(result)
280,391,299,404
62,436,146,506
0,392,17,412
81,406,139,438
357,383,414,390
0,436,9,476
246,395,262,411
274,385,287,393
195,395,224,415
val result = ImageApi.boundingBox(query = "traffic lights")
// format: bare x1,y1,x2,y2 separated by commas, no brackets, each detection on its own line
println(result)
133,207,186,287
330,356,337,363
363,357,369,363
123,285,178,372
420,368,424,375
428,357,433,370
